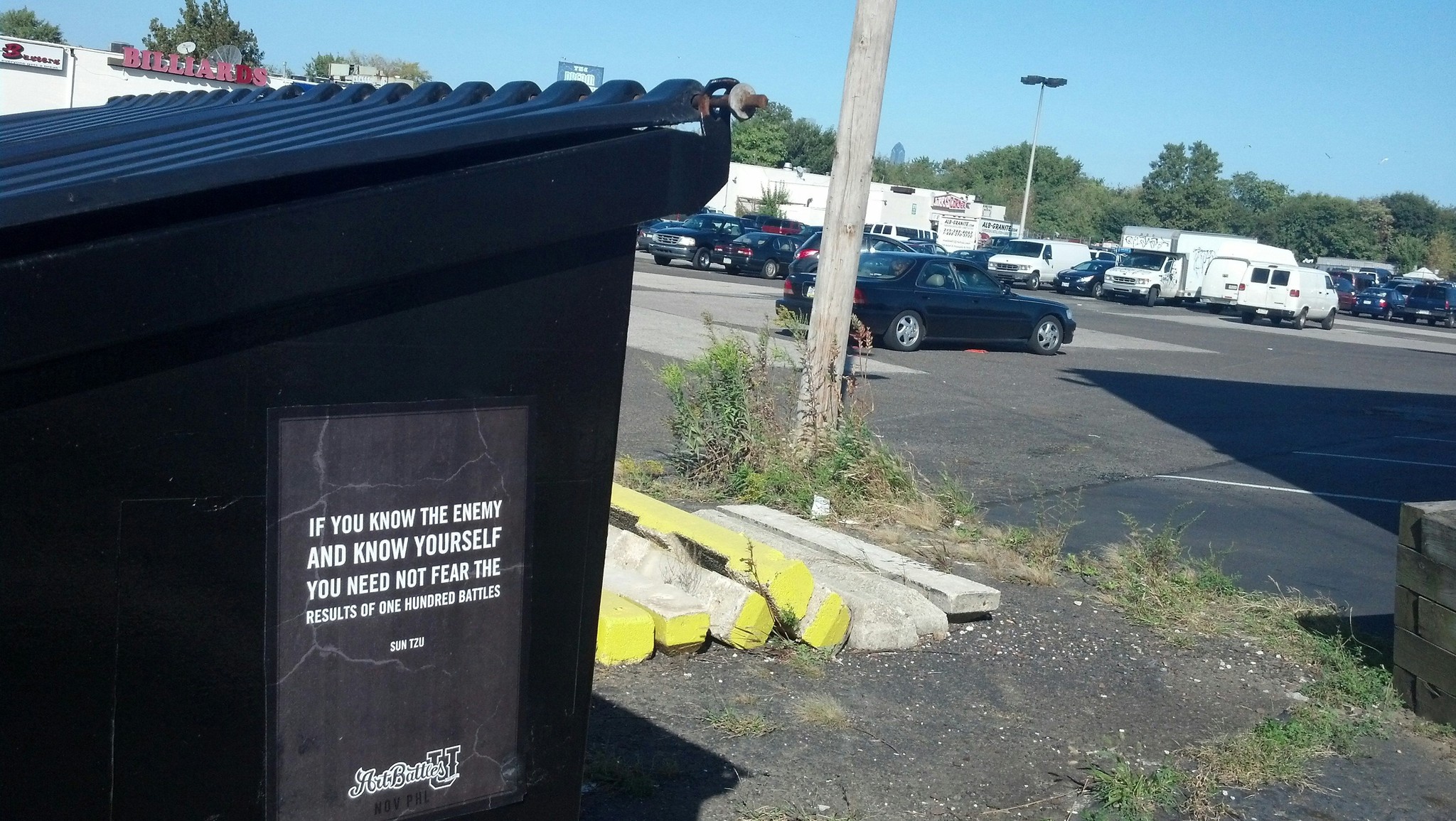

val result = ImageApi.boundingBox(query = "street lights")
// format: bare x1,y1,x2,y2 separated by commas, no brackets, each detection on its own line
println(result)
1018,75,1067,240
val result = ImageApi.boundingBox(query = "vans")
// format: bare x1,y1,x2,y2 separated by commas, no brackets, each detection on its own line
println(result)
1196,256,1281,318
986,238,1092,291
1236,263,1339,331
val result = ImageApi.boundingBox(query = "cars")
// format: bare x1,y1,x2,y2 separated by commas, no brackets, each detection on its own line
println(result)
1258,245,1438,321
1053,260,1116,297
1089,250,1117,262
636,206,1021,321
775,251,1077,355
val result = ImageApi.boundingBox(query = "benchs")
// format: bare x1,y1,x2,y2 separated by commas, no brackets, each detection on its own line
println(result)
743,238,765,244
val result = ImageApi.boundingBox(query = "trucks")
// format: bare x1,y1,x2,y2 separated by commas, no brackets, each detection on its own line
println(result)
1101,225,1258,307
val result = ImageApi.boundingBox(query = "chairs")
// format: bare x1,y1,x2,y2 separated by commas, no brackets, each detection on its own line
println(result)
924,274,946,289
729,226,739,234
781,244,789,250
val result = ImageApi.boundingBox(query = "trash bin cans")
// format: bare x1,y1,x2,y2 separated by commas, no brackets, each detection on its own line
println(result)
0,74,768,821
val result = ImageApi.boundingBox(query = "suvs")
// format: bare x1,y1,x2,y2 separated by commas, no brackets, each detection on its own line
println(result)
1403,280,1456,328
